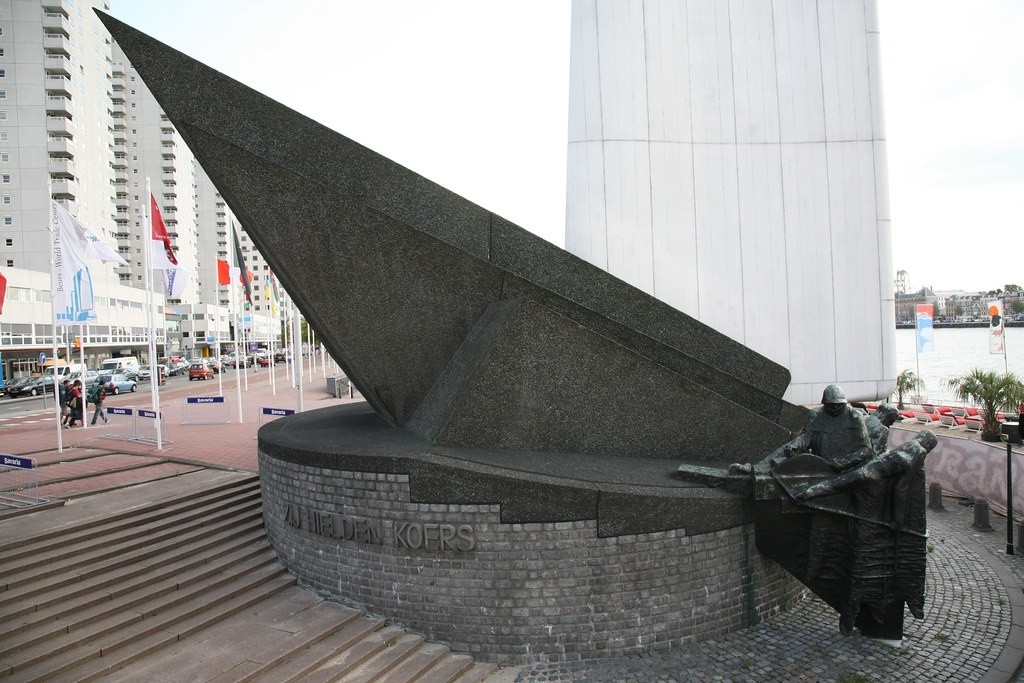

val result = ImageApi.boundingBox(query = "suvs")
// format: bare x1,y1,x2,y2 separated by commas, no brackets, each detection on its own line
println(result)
59,369,98,391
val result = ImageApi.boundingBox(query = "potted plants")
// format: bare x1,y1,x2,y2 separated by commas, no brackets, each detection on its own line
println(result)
940,365,1024,442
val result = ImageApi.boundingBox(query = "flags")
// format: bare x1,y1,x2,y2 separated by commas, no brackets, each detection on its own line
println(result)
218,259,254,286
987,300,1004,354
52,199,130,327
151,192,190,300
917,304,935,353
266,269,280,313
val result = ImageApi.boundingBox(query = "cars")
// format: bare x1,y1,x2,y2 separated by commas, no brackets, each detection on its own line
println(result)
0,376,34,398
6,375,54,397
139,343,327,381
108,368,142,383
92,373,138,395
189,362,215,381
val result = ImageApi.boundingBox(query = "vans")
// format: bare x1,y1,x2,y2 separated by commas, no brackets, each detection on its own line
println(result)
43,363,88,379
97,357,138,376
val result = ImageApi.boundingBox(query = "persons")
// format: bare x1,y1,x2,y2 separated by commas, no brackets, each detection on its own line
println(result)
59,380,71,425
65,380,83,429
91,380,111,427
865,403,899,454
783,384,872,473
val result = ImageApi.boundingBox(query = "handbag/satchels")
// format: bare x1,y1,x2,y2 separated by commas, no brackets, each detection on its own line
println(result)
66,395,76,407
86,386,99,403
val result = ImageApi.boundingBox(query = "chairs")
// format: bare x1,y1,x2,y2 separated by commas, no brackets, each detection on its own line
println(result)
866,403,1006,434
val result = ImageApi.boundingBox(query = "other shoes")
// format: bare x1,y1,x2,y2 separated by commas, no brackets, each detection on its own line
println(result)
74,421,77,425
91,423,97,427
65,423,70,429
106,419,111,424
